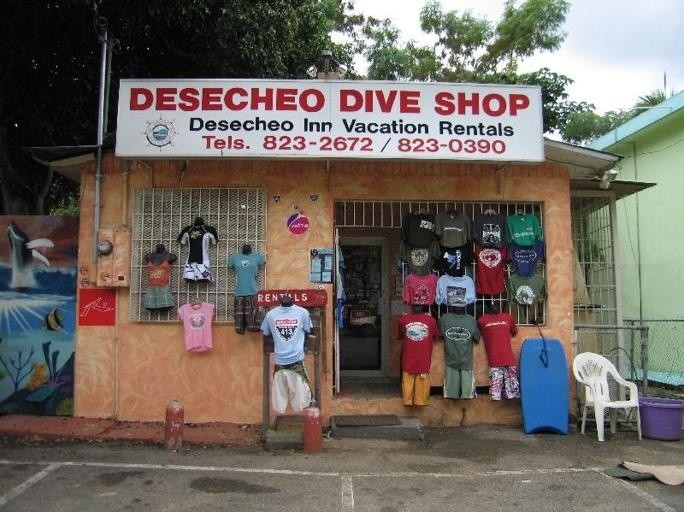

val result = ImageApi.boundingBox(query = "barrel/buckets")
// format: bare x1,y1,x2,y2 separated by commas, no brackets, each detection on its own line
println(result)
639,397,684,441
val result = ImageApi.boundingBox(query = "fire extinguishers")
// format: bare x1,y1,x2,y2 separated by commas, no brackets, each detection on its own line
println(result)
303,402,322,454
165,400,184,449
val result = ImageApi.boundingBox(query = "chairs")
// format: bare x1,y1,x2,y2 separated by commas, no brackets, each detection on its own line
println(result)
572,351,642,441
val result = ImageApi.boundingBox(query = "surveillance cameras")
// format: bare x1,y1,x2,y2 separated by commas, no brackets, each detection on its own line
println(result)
599,169,617,190
